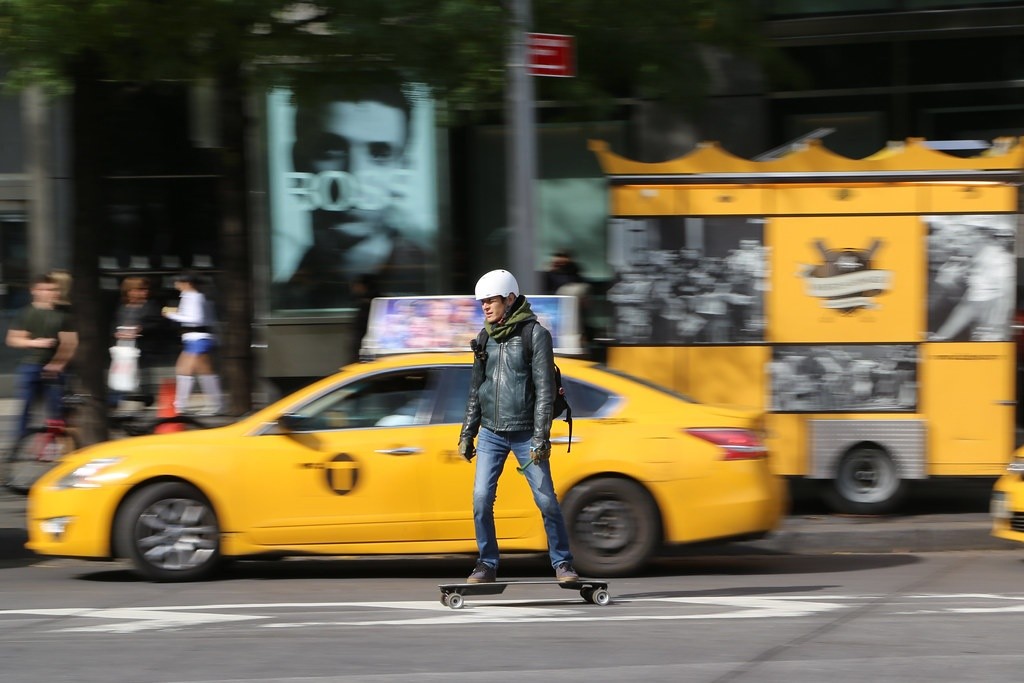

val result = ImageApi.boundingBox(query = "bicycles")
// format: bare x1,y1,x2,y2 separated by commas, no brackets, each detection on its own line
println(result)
3,386,211,498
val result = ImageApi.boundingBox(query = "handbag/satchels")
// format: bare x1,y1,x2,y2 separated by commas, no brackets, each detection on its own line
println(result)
105,345,142,392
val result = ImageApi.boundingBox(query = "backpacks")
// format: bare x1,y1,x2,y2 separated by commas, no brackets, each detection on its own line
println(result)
479,319,573,453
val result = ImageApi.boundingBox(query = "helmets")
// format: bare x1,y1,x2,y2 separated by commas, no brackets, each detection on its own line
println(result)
475,269,520,300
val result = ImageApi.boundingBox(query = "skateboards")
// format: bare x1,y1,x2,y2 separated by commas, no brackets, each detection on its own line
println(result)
437,578,613,610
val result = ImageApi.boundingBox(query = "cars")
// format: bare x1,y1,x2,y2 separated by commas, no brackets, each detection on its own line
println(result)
989,447,1024,547
24,294,784,585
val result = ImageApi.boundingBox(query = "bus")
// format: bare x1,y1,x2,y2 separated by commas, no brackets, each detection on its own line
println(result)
592,117,1024,512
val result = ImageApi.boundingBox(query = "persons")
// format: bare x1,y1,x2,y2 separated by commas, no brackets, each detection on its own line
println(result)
458,269,580,584
271,78,446,308
105,275,154,412
4,273,82,459
158,271,227,416
338,273,384,367
544,250,585,296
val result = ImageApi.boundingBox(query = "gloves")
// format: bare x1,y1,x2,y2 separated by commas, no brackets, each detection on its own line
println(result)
458,441,477,463
529,448,551,464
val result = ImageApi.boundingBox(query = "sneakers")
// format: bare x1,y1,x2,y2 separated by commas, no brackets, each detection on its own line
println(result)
555,562,580,581
466,562,497,583
